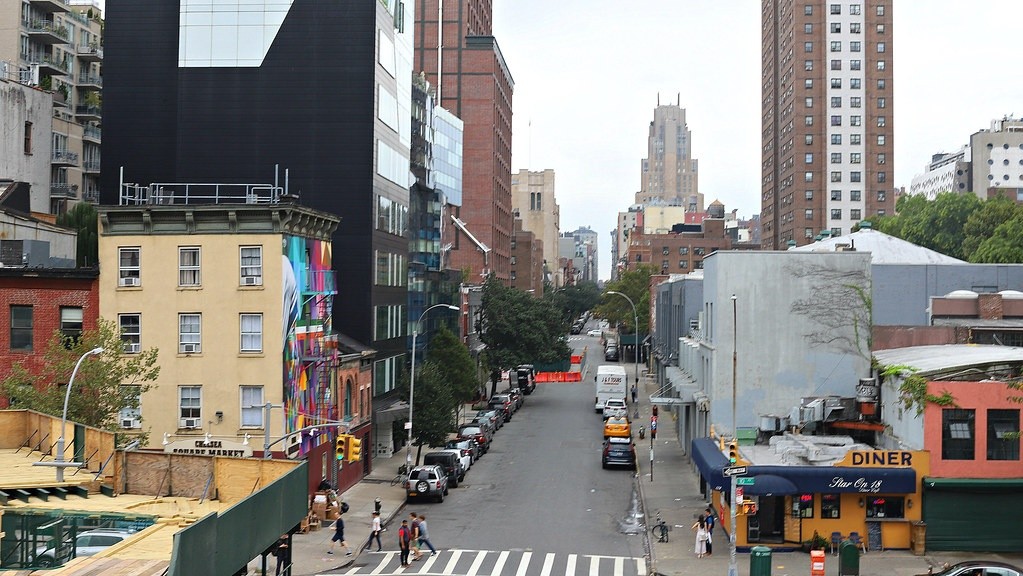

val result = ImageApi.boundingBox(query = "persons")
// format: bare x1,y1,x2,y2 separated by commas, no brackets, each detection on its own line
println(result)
326,511,353,558
630,384,636,404
275,534,290,576
364,511,383,552
652,403,658,417
398,512,437,568
691,508,715,558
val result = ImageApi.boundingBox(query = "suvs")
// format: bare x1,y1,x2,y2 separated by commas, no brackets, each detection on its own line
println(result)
405,464,449,503
423,452,464,487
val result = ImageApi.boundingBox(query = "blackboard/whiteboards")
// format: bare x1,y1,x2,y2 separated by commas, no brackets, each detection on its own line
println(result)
866,521,883,552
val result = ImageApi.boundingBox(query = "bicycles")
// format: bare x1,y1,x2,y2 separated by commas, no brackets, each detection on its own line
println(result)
390,464,408,488
651,510,669,544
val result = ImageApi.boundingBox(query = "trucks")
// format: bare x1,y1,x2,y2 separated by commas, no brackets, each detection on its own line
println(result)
594,365,627,411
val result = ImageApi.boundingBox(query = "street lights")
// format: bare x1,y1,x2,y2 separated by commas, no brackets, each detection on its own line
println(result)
603,291,639,399
405,303,462,478
52,346,108,485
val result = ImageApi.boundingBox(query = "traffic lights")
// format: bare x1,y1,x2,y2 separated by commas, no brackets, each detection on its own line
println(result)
729,440,739,464
335,436,345,461
741,501,757,516
349,438,362,462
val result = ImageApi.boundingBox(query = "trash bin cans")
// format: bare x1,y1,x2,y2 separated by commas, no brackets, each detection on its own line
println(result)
749,545,773,576
838,540,861,576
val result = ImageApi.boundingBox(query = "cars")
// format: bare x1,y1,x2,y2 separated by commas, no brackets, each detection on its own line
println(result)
604,416,633,440
456,386,524,453
442,449,472,473
601,435,636,471
603,333,619,361
571,310,592,335
444,440,480,465
587,330,602,337
602,399,629,421
913,558,1023,576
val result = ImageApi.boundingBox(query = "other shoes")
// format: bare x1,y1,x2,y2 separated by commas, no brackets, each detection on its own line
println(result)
401,564,407,568
365,548,371,551
431,552,437,556
327,551,334,554
412,552,423,561
345,552,352,557
405,564,411,567
376,548,383,552
697,553,712,559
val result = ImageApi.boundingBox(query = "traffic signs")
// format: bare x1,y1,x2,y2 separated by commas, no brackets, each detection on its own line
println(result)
721,465,748,478
